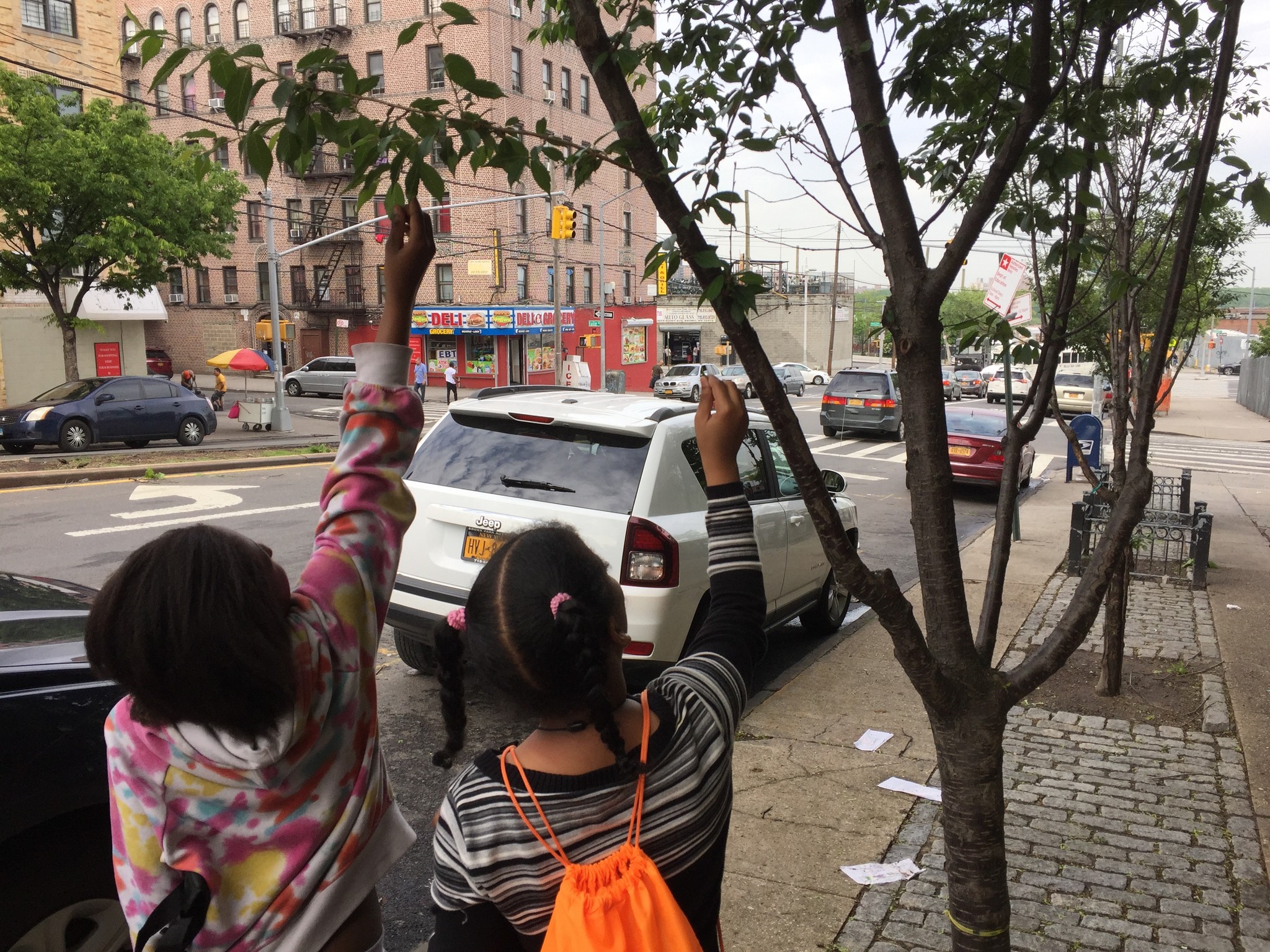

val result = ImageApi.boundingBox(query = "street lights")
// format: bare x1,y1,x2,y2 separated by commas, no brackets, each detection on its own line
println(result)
803,268,818,367
1232,258,1256,359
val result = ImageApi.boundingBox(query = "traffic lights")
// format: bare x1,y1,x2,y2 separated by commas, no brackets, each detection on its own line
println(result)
1209,342,1216,349
562,206,577,238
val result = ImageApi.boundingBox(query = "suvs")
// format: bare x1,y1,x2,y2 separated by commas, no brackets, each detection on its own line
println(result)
384,383,861,694
653,361,723,403
721,364,759,399
820,367,905,442
145,346,174,380
987,366,1037,405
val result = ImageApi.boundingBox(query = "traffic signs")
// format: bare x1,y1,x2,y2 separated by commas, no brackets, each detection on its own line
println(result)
1216,333,1227,336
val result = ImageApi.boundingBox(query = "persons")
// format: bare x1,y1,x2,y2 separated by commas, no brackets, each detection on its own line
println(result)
181,370,195,390
443,362,460,405
687,346,693,364
1173,354,1177,365
84,197,436,952
651,360,665,382
414,357,427,404
210,368,226,411
262,348,267,355
426,375,769,952
693,345,698,364
476,358,483,373
665,345,672,366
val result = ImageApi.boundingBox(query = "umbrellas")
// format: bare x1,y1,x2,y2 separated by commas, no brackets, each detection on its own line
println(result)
206,347,274,398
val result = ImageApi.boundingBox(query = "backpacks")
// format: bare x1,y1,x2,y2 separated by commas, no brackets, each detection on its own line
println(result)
502,689,724,952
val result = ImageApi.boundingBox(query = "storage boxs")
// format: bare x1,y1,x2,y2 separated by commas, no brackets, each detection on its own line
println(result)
567,355,581,362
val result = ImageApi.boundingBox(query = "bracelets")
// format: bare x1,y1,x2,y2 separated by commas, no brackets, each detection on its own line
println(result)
423,379,425,381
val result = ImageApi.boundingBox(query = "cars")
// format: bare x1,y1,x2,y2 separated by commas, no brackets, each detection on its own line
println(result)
283,355,356,397
905,407,1037,501
771,366,806,397
0,568,132,951
1102,379,1115,409
951,354,955,365
942,370,988,402
1045,370,1111,421
1217,359,1241,376
0,375,218,454
770,362,831,386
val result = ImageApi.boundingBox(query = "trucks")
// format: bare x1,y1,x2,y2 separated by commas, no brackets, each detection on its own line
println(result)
954,335,992,372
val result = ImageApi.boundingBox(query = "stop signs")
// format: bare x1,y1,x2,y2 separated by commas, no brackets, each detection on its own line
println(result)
1220,337,1223,345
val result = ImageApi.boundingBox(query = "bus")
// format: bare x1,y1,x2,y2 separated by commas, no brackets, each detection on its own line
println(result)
1054,343,1111,375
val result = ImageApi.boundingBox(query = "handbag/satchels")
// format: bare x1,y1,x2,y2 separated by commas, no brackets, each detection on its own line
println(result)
134,872,210,952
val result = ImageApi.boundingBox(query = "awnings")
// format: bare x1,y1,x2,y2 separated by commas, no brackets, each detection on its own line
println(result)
64,280,168,320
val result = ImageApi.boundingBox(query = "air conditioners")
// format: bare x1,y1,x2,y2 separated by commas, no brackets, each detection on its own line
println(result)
209,98,225,109
224,294,240,303
544,161,551,172
624,296,631,303
168,294,184,303
206,34,220,43
290,229,302,238
543,90,556,102
510,5,520,16
